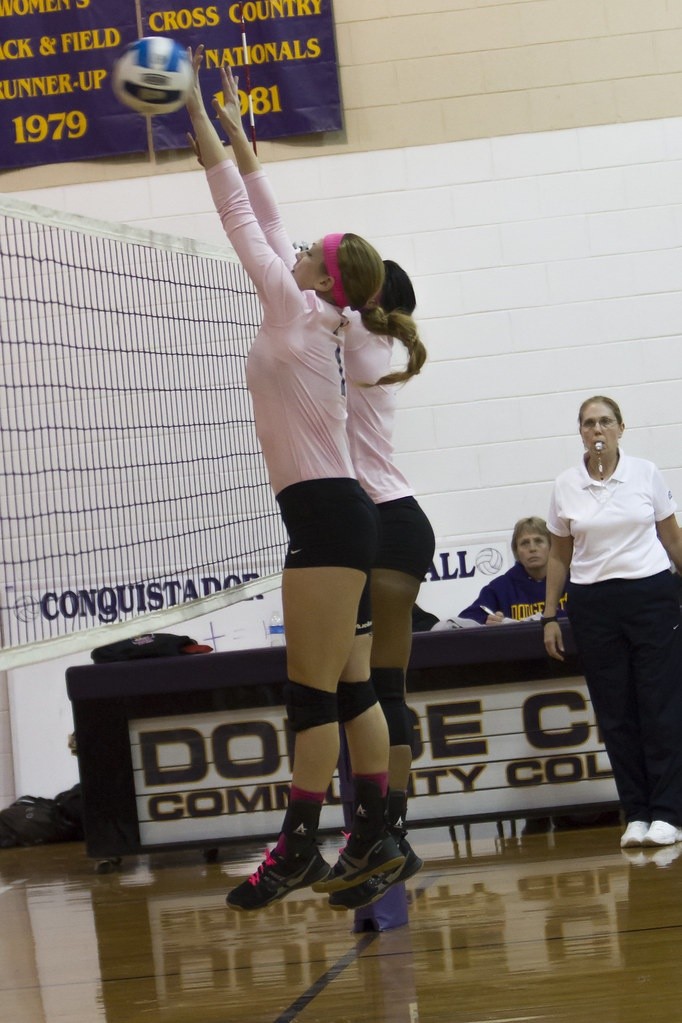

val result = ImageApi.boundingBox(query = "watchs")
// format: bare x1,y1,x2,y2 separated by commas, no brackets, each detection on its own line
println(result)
540,616,558,626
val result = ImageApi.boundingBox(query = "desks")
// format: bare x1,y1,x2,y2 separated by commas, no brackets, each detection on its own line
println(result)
66,617,625,872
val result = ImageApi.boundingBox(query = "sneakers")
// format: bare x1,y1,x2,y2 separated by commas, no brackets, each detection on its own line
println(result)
620,820,649,848
642,820,682,846
328,828,424,911
225,836,330,912
313,833,407,894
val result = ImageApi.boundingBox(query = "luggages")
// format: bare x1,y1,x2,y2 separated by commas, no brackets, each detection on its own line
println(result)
0,782,75,847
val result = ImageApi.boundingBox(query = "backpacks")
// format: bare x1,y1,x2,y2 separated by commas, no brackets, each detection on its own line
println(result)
52,782,86,839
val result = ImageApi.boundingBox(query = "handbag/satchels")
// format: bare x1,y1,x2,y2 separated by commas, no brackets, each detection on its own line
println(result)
91,633,213,664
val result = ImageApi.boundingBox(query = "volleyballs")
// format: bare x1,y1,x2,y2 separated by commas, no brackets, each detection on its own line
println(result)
111,37,195,116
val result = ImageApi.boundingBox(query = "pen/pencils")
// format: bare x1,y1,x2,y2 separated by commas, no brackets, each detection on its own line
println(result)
479,605,495,616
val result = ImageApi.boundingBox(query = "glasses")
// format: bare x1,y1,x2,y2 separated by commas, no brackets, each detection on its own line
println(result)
580,416,617,433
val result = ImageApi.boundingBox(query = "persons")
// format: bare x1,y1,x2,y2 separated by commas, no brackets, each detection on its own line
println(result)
185,45,427,913
187,132,435,912
457,517,570,626
543,395,682,848
622,849,682,970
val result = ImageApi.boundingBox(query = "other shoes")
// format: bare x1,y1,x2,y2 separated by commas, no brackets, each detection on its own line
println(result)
521,817,582,834
601,811,619,828
